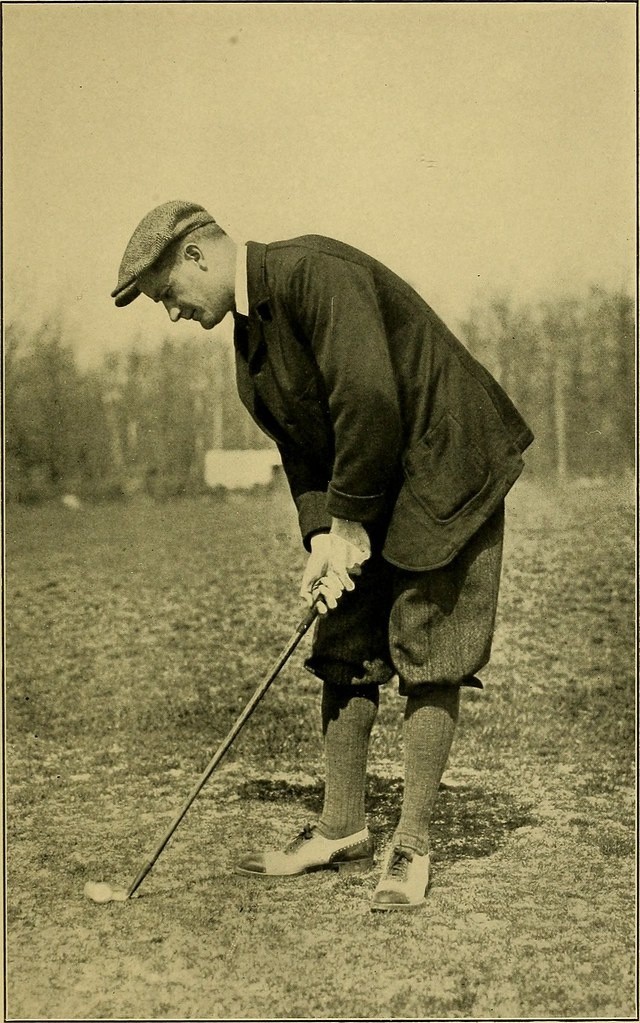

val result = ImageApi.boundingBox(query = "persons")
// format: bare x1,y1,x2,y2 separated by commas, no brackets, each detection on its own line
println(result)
110,200,535,912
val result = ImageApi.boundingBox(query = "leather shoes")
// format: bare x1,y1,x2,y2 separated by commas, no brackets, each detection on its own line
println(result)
370,846,430,911
234,822,374,878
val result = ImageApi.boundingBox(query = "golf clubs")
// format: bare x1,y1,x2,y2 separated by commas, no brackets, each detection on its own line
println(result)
84,594,323,907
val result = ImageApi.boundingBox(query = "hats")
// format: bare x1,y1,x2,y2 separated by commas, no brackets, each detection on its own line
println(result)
111,200,216,308
